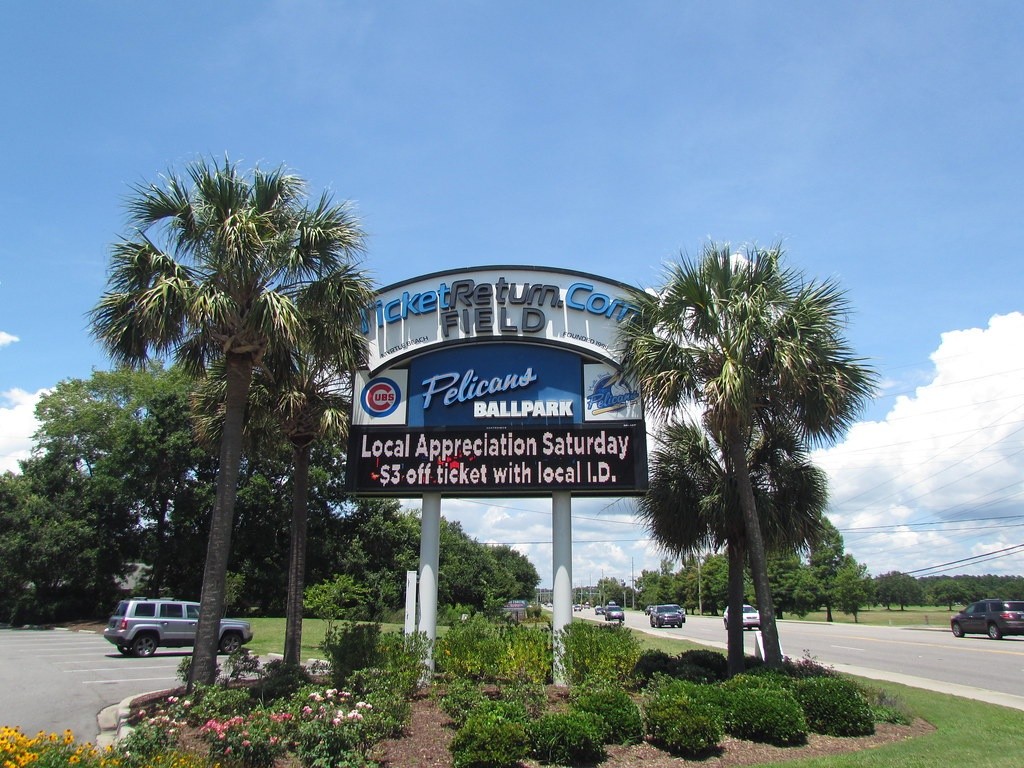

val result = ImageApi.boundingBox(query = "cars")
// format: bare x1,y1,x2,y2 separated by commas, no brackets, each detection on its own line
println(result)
544,601,552,607
644,603,686,629
604,604,625,621
723,603,760,630
574,604,590,612
595,607,605,615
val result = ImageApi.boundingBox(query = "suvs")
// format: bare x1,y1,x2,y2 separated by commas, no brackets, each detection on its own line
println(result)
103,596,255,658
949,599,1024,640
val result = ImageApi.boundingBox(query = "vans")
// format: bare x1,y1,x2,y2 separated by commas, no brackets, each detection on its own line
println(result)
608,601,617,607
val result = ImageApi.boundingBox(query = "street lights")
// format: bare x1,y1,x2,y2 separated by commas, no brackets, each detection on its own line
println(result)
685,565,703,618
